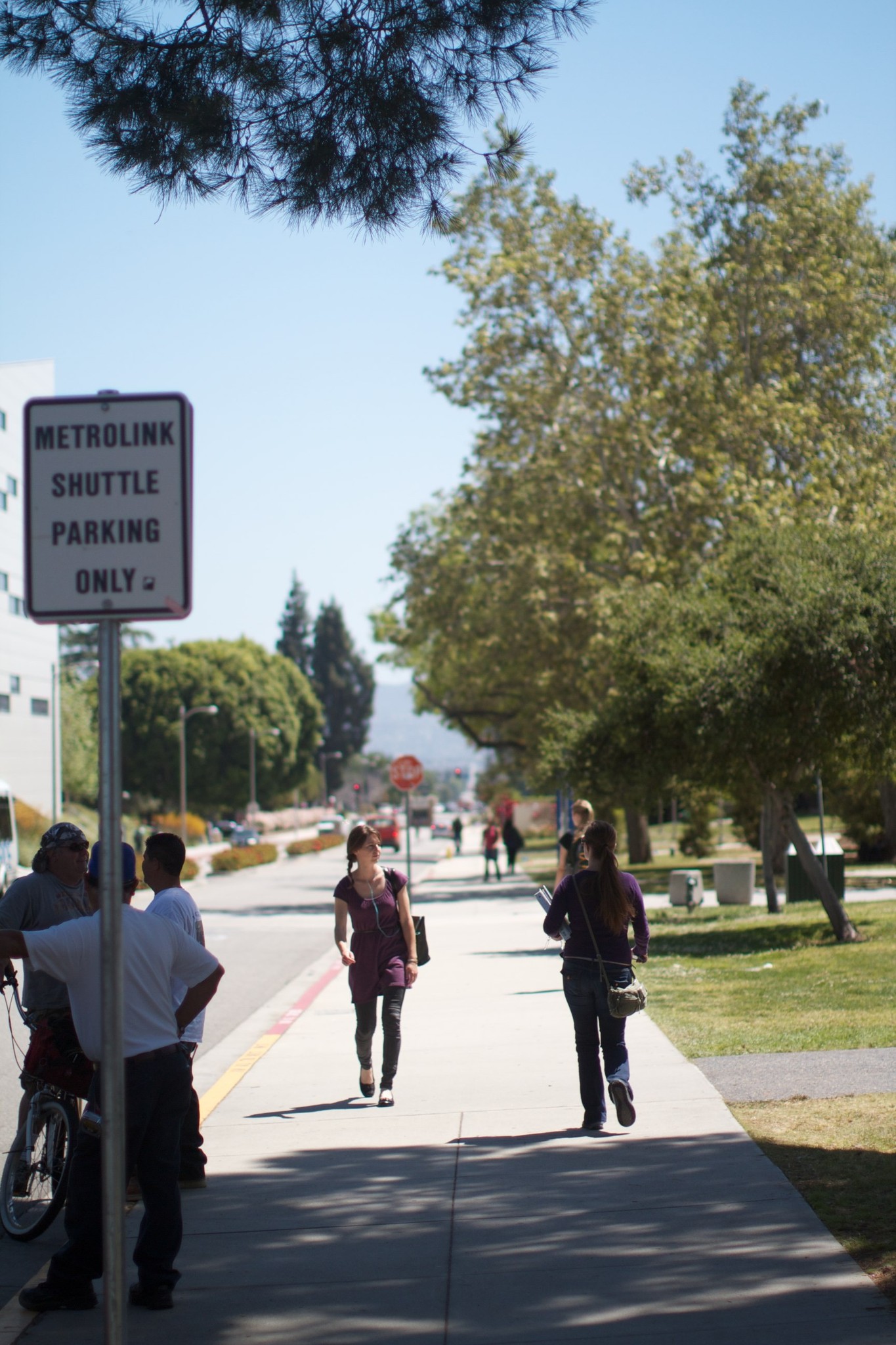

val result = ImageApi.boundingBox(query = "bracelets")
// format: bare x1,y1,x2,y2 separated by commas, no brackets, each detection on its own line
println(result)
407,960,418,962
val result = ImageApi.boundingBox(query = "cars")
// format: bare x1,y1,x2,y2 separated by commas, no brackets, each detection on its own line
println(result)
319,815,347,839
431,818,454,839
409,804,431,826
363,814,401,853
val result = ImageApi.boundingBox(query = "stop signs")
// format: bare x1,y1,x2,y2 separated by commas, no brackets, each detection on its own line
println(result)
388,754,426,792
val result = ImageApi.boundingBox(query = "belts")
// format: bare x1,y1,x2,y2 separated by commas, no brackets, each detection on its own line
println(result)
93,1044,178,1072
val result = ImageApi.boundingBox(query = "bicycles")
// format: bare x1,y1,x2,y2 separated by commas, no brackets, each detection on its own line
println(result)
0,975,85,1243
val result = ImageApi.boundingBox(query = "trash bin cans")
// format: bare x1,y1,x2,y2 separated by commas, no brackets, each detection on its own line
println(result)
787,833,845,901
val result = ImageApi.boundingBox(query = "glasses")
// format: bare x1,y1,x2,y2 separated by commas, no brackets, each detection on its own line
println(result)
581,836,590,843
55,841,89,852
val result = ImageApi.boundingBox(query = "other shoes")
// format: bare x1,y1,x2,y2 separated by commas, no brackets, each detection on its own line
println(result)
582,1121,603,1130
126,1180,142,1201
360,1065,375,1097
178,1175,206,1188
611,1080,636,1127
129,1282,174,1310
19,1281,97,1312
378,1094,394,1106
12,1160,30,1196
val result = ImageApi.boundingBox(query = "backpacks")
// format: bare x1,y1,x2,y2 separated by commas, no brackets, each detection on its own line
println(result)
569,828,589,869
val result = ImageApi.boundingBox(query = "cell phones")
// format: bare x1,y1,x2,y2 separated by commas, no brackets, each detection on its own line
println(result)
632,954,646,962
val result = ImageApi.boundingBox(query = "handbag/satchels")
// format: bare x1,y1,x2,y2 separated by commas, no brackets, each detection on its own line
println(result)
607,979,648,1019
381,866,430,966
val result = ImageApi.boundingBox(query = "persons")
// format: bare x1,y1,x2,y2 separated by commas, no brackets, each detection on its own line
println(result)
482,819,501,880
125,833,207,1203
134,831,143,854
333,825,419,1107
553,799,594,951
0,841,224,1313
543,820,650,1129
503,817,517,875
142,818,164,835
453,819,462,843
0,822,100,1197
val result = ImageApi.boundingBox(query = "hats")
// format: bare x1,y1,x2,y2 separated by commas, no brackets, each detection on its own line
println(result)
88,840,135,881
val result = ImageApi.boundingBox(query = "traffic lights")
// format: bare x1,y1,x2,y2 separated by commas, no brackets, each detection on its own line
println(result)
352,782,361,794
454,767,463,779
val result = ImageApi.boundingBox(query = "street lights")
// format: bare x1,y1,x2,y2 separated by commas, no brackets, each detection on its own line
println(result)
243,727,283,811
321,750,343,806
177,703,221,849
